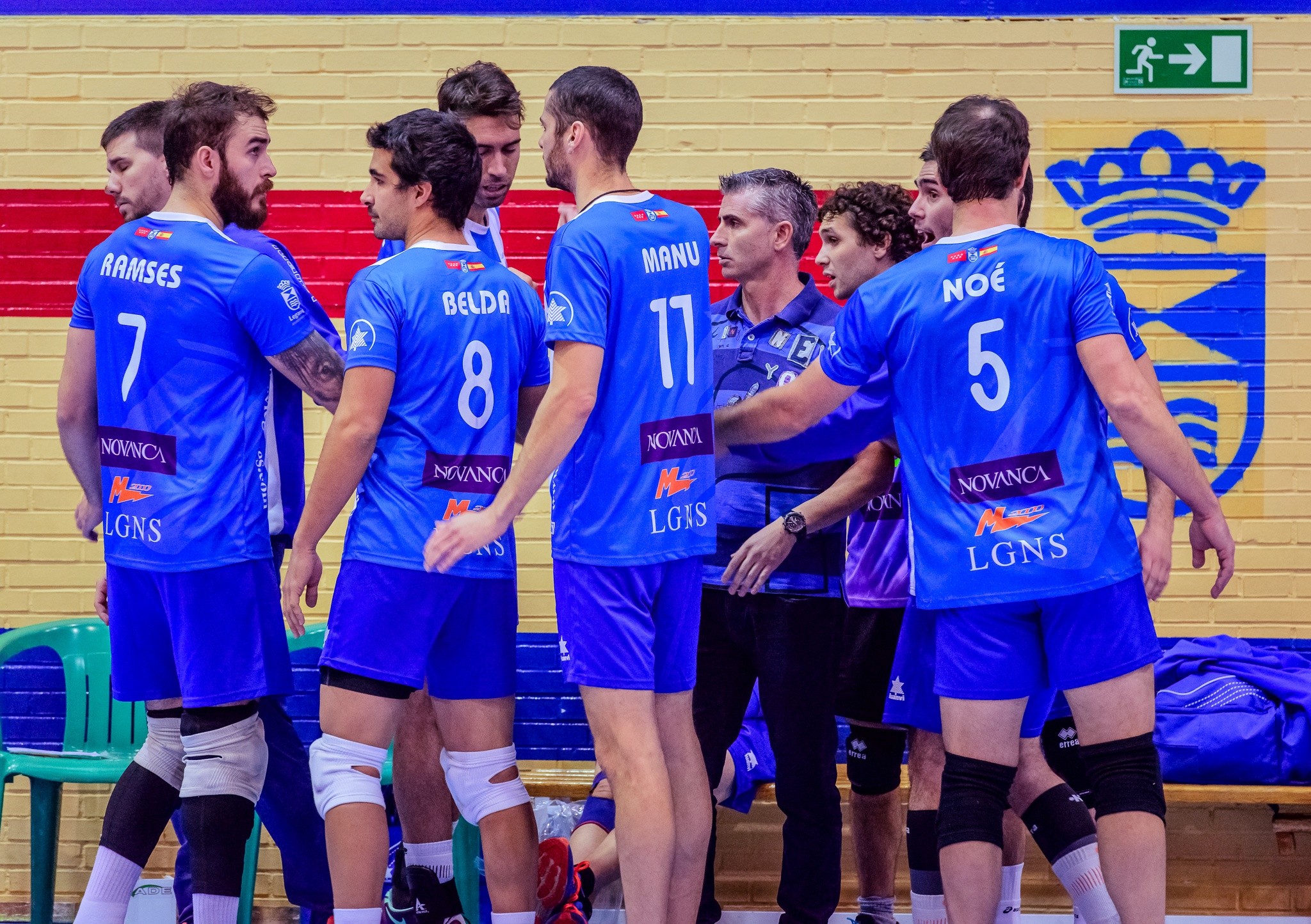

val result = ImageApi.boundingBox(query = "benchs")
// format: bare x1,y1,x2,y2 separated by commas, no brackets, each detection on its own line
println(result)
515,760,1311,807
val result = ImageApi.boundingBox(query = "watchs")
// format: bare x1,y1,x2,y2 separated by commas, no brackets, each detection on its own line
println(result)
780,507,809,544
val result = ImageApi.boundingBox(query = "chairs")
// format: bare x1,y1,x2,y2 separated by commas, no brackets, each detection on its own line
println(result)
238,621,395,924
0,611,149,924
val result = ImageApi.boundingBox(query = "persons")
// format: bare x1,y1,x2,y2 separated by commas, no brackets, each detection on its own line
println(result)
58,60,1236,924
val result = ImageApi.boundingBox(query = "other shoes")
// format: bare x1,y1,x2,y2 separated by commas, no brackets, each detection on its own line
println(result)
536,836,597,924
379,866,446,924
